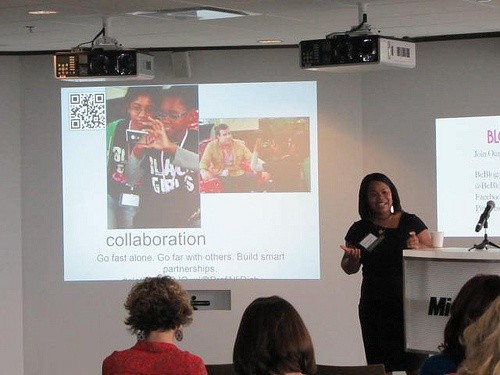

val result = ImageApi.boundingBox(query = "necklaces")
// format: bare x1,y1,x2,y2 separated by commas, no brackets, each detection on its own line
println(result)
378,216,395,234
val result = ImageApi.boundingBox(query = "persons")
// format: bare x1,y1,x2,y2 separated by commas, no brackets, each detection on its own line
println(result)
125,85,198,229
102,275,209,375
339,173,433,375
106,86,163,228
233,296,317,375
199,123,309,193
418,274,500,375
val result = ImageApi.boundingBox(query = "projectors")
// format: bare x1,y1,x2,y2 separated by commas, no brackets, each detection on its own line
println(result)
300,36,416,71
54,51,154,82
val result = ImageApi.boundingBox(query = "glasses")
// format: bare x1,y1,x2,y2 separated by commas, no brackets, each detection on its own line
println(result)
129,104,155,114
156,110,189,121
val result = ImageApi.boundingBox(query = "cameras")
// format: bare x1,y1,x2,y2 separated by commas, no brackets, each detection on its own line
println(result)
126,130,149,144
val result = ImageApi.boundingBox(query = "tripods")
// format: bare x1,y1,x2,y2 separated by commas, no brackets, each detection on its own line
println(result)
468,219,500,252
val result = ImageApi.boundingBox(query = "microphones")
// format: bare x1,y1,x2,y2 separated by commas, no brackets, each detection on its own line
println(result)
475,201,495,232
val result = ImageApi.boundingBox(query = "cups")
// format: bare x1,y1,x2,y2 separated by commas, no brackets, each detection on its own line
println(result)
430,232,444,248
